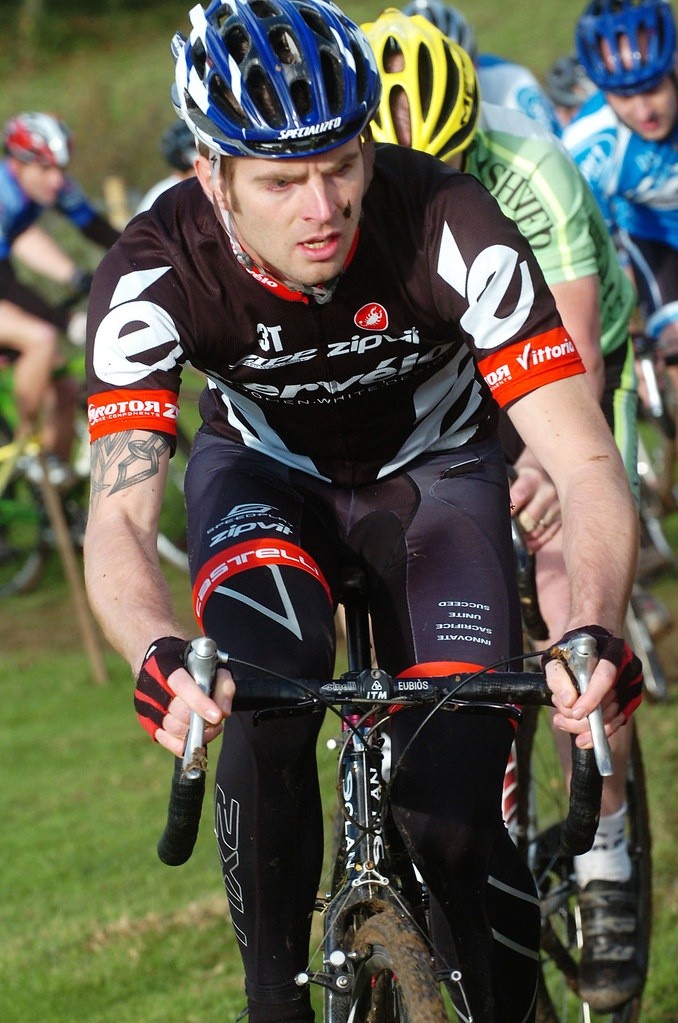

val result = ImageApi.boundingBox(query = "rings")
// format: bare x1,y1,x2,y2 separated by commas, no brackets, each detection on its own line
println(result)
539,518,548,531
519,510,537,534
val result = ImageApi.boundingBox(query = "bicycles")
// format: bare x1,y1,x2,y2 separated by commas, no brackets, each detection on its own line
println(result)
155,633,614,1023
0,407,193,599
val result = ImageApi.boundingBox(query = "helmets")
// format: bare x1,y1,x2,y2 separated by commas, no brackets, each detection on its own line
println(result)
402,0,476,58
165,118,199,172
573,0,677,97
547,54,599,106
358,7,481,165
5,111,74,169
170,0,381,161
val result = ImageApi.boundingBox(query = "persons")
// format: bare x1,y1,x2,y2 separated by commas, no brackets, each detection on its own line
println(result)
360,0,678,1008
0,109,122,486
83,0,643,1023
134,119,198,217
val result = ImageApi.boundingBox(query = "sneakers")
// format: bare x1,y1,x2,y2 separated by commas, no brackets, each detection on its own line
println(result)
10,440,69,485
632,590,672,639
567,847,648,1011
72,426,91,478
